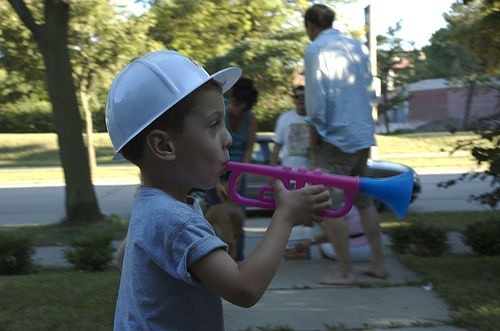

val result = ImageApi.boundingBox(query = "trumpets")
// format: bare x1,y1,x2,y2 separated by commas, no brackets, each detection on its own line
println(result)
225,158,416,221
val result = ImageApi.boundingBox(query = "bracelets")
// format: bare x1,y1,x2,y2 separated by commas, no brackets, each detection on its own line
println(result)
310,237,315,243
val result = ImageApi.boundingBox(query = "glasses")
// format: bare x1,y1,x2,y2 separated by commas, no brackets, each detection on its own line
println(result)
294,94,304,99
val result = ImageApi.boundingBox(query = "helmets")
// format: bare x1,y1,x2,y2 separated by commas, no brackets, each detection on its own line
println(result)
105,50,242,162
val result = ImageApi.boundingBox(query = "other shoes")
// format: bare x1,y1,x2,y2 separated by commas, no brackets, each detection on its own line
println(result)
284,250,309,260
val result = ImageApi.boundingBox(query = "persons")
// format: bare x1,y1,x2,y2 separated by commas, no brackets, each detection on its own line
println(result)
271,85,310,169
205,78,258,261
295,201,373,261
106,50,333,330
304,4,389,283
